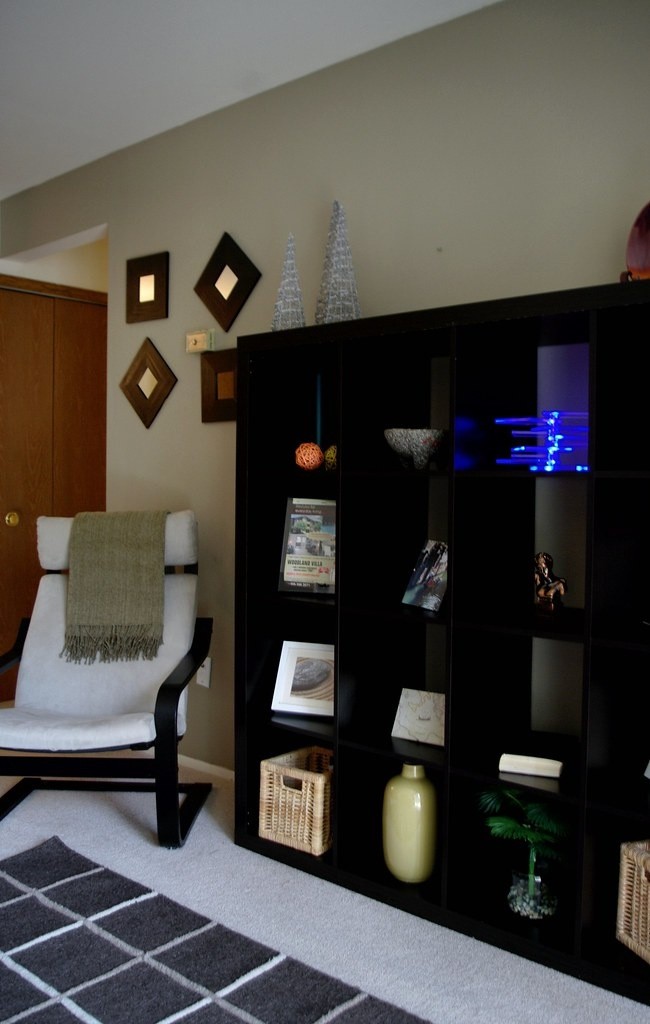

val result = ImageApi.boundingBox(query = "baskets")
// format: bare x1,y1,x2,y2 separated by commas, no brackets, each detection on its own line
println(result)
616,840,650,964
259,746,334,856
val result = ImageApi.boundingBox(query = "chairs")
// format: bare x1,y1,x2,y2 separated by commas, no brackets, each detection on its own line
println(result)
0,507,213,850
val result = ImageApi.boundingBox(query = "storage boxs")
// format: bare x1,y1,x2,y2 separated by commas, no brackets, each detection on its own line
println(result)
258,745,334,855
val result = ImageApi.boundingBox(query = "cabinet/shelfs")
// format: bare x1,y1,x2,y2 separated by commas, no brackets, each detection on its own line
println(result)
234,279,649,1006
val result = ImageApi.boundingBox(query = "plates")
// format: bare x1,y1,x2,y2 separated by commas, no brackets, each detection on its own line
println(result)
627,201,650,280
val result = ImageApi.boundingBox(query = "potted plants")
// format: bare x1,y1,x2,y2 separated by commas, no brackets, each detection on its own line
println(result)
479,786,568,920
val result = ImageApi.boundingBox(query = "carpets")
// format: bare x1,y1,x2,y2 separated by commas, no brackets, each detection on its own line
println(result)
0,834,432,1023
